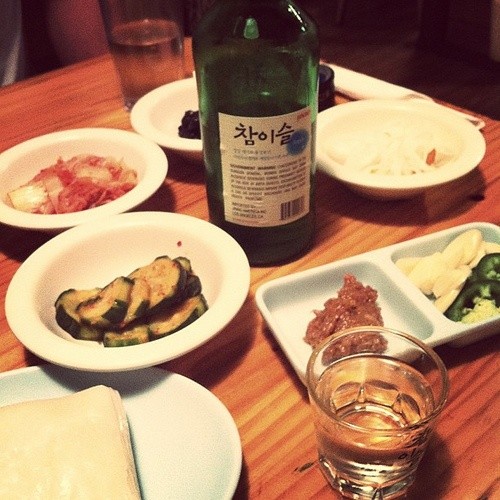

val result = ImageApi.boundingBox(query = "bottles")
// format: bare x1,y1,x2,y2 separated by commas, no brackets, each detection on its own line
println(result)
192,0,320,268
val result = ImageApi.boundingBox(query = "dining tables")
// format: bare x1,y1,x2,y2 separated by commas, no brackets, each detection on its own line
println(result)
0,36,499,500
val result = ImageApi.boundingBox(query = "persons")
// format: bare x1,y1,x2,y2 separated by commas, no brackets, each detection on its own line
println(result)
0,0,109,87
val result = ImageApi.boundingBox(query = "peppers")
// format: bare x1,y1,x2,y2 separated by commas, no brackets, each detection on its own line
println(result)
444,252,500,325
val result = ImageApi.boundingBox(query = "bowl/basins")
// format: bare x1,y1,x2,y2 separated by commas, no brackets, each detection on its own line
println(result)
315,98,486,201
130,78,202,160
5,211,250,371
0,126,168,232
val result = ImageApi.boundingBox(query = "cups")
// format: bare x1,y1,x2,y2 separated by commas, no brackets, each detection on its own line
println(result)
319,64,335,112
306,326,449,500
97,0,186,114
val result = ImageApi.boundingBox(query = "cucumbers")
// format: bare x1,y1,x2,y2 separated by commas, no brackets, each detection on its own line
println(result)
53,256,207,347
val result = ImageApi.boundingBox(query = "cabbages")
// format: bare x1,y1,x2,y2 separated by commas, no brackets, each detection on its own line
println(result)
8,153,136,214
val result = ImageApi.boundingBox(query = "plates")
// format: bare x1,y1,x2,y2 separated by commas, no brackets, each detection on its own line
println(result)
255,221,500,386
0,363,242,500
